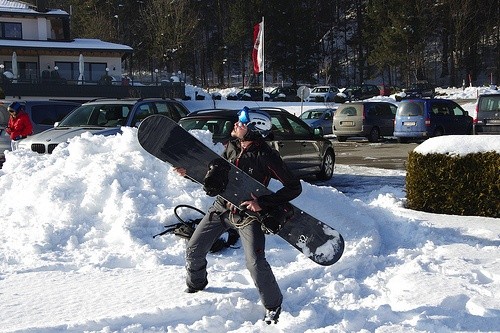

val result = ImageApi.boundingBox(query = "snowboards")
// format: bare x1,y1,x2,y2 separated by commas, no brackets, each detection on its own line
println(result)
136,114,346,267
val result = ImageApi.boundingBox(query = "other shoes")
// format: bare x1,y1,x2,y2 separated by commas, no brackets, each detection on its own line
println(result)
184,288,203,293
263,306,281,324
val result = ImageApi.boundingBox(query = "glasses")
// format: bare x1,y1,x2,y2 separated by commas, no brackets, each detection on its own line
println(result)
238,122,247,131
237,106,250,125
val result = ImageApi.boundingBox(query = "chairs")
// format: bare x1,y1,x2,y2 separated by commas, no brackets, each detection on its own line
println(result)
136,109,149,120
206,122,220,133
99,111,121,125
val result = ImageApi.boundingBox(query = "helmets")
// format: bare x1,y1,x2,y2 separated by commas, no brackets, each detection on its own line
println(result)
243,109,272,138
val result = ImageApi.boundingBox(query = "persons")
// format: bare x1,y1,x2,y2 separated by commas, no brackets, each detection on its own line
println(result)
0,100,10,136
172,106,302,325
5,101,32,151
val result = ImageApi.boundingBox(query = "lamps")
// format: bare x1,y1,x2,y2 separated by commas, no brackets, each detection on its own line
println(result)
0,64,4,68
178,71,181,75
106,68,110,71
154,68,158,73
54,66,58,70
